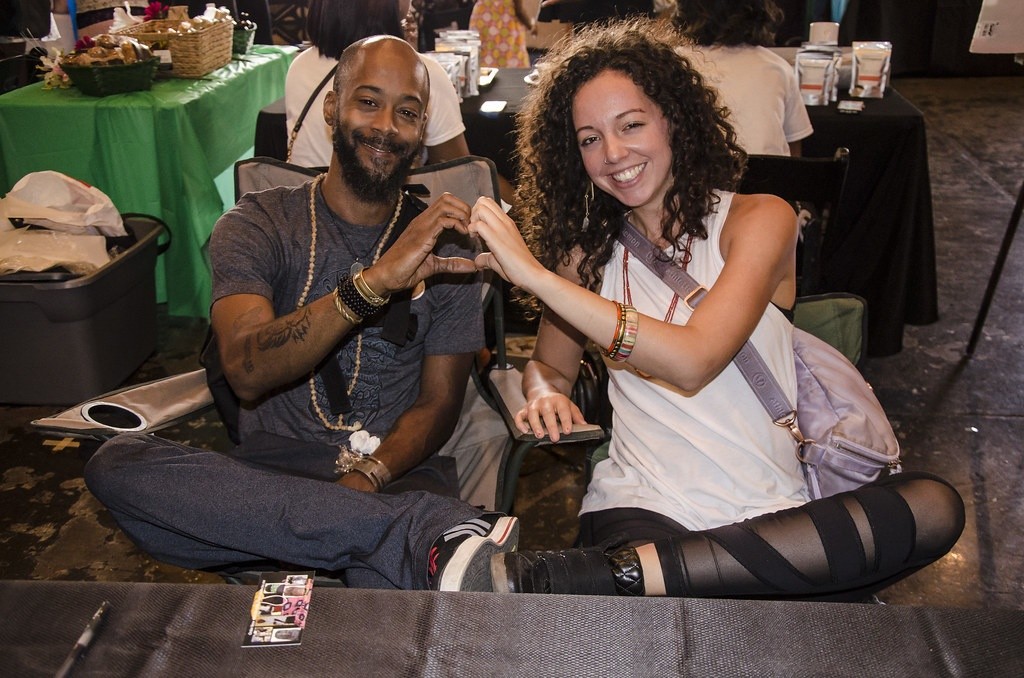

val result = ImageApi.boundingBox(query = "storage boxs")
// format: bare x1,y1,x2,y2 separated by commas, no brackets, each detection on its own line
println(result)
0,213,173,406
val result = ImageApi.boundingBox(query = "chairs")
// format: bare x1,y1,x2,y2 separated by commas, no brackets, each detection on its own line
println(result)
31,156,603,587
734,147,850,297
592,293,870,475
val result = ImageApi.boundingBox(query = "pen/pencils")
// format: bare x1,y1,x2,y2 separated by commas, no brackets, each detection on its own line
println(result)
52,599,110,678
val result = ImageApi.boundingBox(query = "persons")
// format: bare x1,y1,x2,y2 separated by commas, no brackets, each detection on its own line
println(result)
470,0,543,68
469,29,966,600
85,35,520,592
667,0,815,156
285,0,523,215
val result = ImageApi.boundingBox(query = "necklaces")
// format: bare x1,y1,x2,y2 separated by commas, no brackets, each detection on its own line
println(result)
298,173,403,433
623,235,694,380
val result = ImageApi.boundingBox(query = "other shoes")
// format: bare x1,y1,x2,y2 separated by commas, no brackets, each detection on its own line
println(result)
427,515,519,592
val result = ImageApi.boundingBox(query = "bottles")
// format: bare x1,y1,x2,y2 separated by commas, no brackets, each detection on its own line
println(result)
204,3,220,22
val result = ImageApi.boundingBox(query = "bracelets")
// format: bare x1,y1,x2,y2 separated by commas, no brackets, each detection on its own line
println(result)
344,456,392,491
600,300,639,362
333,266,391,324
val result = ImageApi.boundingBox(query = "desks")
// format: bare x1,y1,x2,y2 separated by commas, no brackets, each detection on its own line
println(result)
254,67,944,359
0,45,304,325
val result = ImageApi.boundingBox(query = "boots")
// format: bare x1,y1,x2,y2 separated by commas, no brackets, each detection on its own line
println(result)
490,534,686,597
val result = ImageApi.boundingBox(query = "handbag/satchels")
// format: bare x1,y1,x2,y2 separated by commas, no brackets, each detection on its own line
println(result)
794,326,900,500
0,170,128,277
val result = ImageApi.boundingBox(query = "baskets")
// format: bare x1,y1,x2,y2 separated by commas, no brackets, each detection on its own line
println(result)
59,56,160,97
234,22,257,54
114,19,234,77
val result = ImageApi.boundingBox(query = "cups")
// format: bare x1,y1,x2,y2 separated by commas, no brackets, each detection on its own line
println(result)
810,21,839,45
